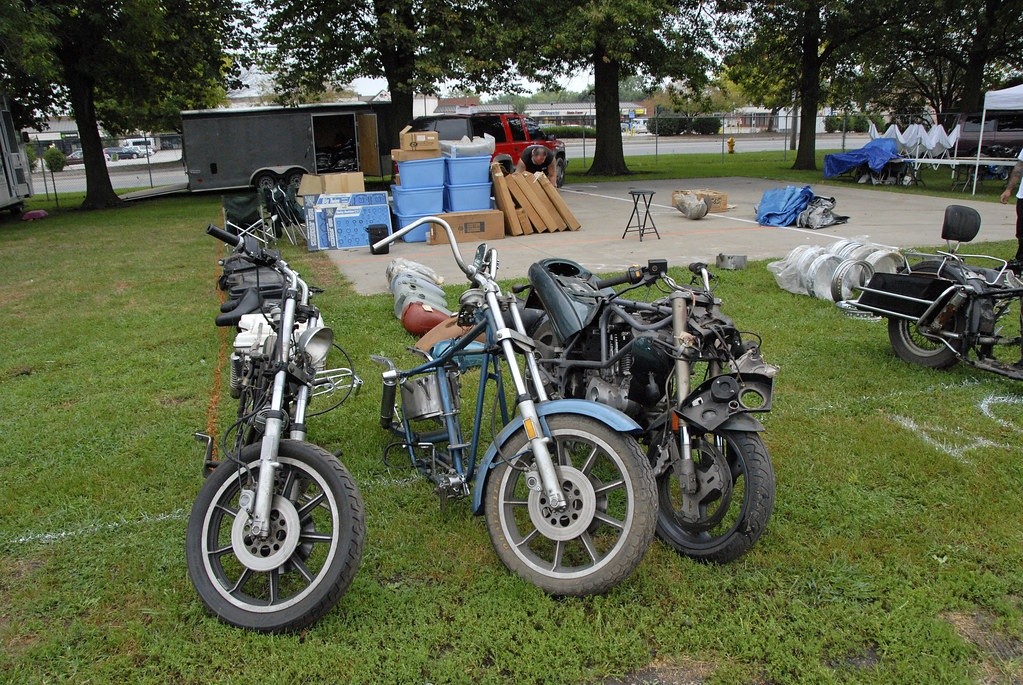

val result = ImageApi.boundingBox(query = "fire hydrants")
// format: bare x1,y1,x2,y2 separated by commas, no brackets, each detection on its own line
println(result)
726,137,735,154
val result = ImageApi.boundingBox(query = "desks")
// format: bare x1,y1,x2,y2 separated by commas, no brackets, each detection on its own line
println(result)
835,157,1019,193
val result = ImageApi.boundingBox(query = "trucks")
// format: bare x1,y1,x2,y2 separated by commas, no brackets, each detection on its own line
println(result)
119,139,155,149
0,93,34,213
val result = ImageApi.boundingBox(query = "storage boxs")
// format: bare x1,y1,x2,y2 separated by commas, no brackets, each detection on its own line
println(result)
672,189,727,211
390,125,581,245
297,172,365,196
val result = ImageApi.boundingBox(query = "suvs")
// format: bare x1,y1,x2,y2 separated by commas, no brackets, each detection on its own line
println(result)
391,112,569,188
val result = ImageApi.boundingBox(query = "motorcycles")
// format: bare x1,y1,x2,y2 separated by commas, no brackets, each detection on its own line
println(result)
833,204,1023,383
500,257,776,563
185,224,366,633
372,216,660,597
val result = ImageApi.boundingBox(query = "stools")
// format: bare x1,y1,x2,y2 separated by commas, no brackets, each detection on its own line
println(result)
622,190,660,242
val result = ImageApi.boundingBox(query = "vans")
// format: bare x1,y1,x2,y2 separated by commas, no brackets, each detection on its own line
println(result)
943,111,1023,171
104,147,139,160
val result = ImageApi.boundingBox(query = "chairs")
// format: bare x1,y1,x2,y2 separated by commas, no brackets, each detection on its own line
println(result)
221,182,308,253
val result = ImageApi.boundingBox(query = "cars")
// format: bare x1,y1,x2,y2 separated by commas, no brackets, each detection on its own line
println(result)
125,146,152,158
539,124,555,127
66,151,84,165
160,142,172,150
621,123,628,132
632,125,647,134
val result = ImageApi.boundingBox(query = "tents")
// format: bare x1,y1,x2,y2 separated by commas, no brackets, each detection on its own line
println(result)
868,124,961,179
972,84,1023,195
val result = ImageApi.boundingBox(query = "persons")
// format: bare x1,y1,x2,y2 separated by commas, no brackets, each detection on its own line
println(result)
515,145,557,190
1000,147,1023,260
